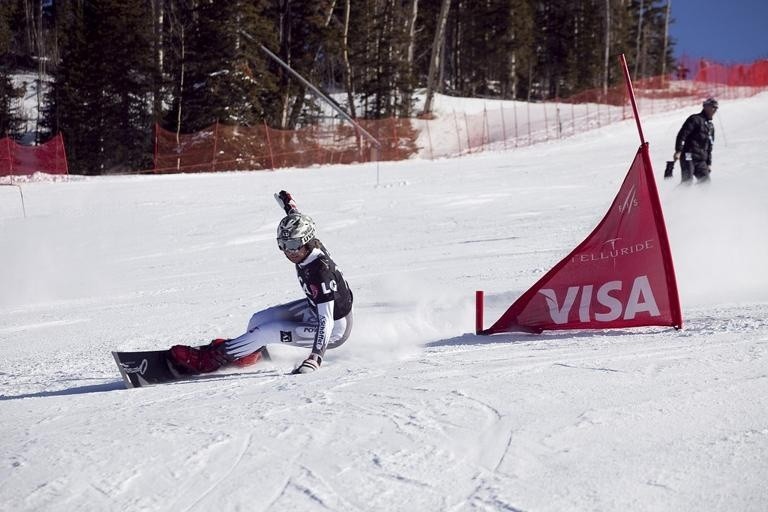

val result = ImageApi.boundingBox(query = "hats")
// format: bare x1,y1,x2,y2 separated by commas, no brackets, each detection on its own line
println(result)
702,98,718,107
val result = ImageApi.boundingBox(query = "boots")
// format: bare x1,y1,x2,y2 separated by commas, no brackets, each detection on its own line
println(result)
169,338,230,374
211,339,265,368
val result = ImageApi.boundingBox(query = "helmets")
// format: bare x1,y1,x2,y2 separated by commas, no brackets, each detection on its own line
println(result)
276,213,316,251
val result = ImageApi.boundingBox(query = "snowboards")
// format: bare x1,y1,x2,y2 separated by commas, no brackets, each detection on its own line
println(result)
111,340,272,388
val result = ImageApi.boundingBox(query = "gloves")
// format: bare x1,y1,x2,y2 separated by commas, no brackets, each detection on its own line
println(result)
274,190,297,215
296,352,323,374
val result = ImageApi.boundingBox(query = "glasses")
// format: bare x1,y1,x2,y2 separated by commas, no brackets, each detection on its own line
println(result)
276,233,311,252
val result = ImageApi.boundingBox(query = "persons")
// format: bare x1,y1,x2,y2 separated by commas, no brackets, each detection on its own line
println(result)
169,190,353,374
674,97,719,184
